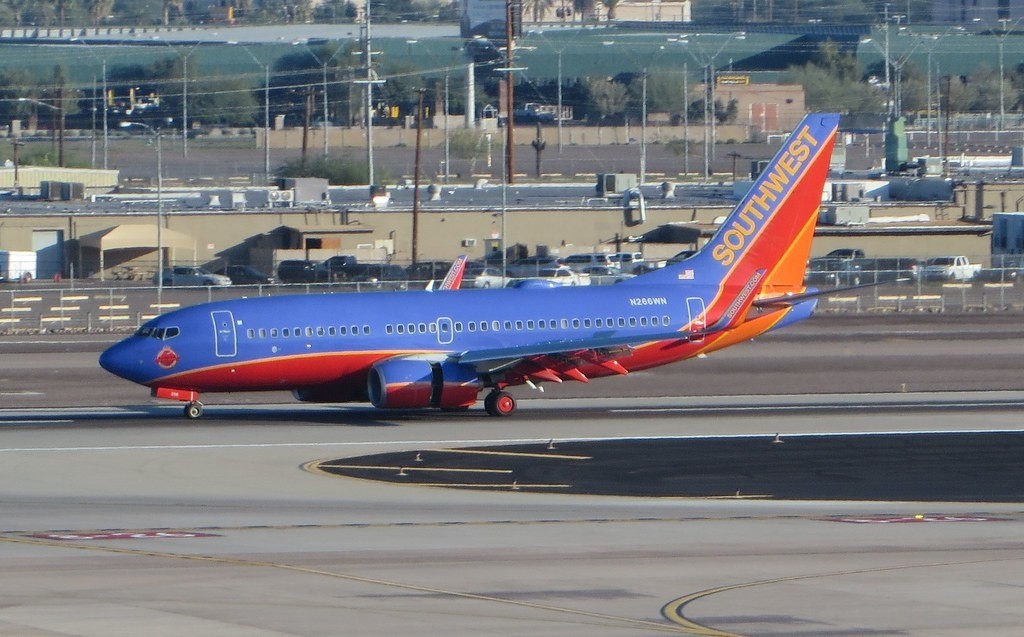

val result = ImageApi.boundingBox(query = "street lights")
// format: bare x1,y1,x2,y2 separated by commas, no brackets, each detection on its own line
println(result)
18,97,67,168
404,33,483,187
118,121,162,317
150,33,209,162
662,30,747,181
69,35,127,168
600,38,678,183
292,35,362,163
534,23,593,155
858,22,965,158
227,40,303,181
973,15,1024,131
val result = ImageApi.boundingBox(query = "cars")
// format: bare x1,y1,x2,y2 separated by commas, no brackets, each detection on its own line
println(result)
450,250,704,289
406,259,460,280
216,263,276,286
277,254,410,291
850,256,921,285
151,265,233,289
809,247,865,286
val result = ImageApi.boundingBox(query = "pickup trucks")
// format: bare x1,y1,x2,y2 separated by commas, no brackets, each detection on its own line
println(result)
922,256,983,283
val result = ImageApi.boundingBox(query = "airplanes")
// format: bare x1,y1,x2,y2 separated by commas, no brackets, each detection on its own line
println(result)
97,113,842,417
